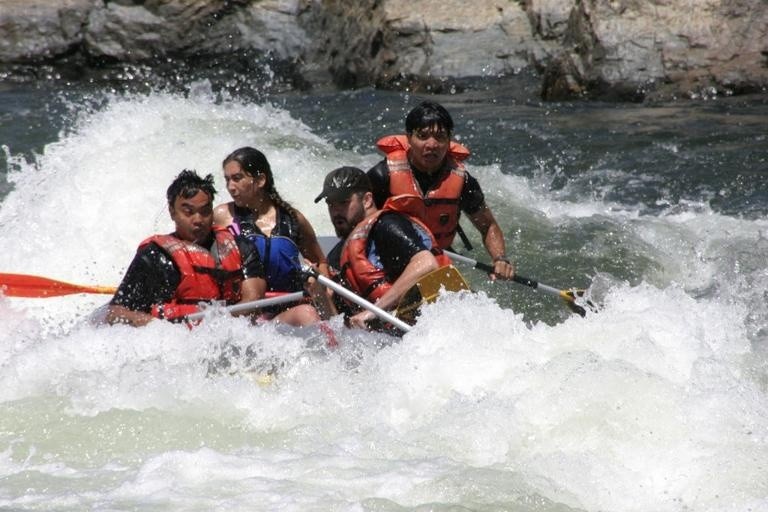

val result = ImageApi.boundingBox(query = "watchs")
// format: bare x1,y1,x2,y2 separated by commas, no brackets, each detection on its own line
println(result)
493,254,511,265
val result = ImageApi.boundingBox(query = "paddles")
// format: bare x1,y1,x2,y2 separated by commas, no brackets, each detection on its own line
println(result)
443,250,598,317
0,273,118,298
369,265,468,327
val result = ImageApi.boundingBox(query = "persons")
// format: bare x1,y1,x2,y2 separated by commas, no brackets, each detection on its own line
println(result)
306,165,438,337
364,101,515,281
214,147,329,293
103,168,320,328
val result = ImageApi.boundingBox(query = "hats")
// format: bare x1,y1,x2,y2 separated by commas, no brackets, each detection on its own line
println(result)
315,167,373,203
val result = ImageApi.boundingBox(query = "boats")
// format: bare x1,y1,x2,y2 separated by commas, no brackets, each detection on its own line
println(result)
77,235,402,385
